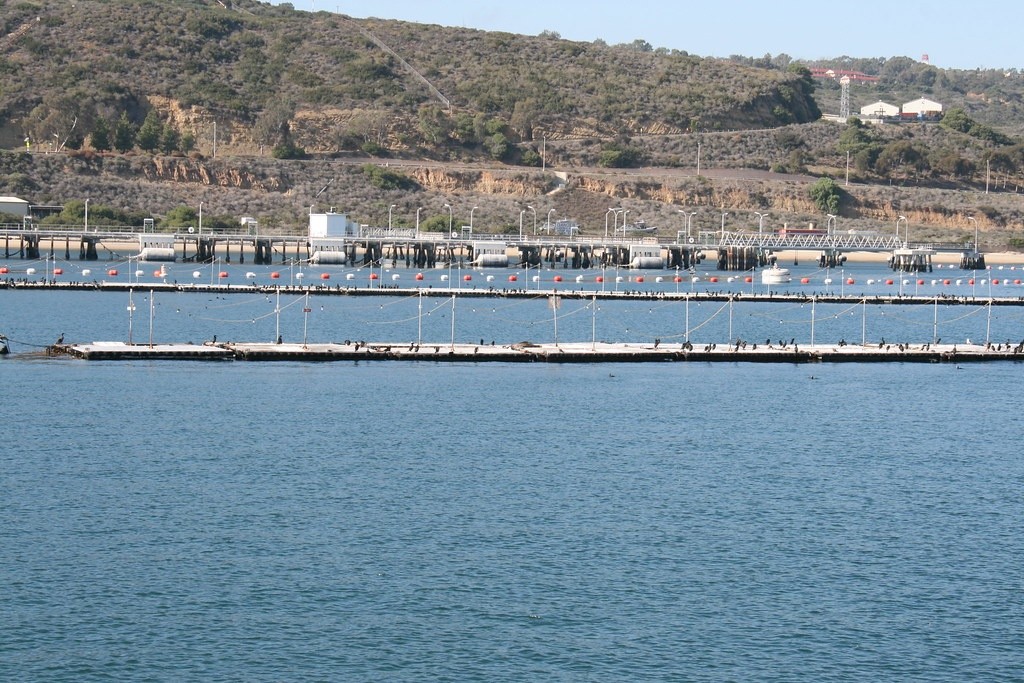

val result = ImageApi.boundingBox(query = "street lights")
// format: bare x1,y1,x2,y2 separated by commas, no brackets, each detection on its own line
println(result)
547,208,555,236
721,213,729,243
519,210,526,241
896,215,909,250
469,206,479,239
388,204,397,234
444,203,452,238
415,207,423,239
845,150,851,185
605,207,631,237
677,209,687,237
967,217,977,252
198,202,204,234
212,121,218,159
83,199,92,232
527,205,537,236
755,212,769,243
689,212,698,236
826,213,837,247
